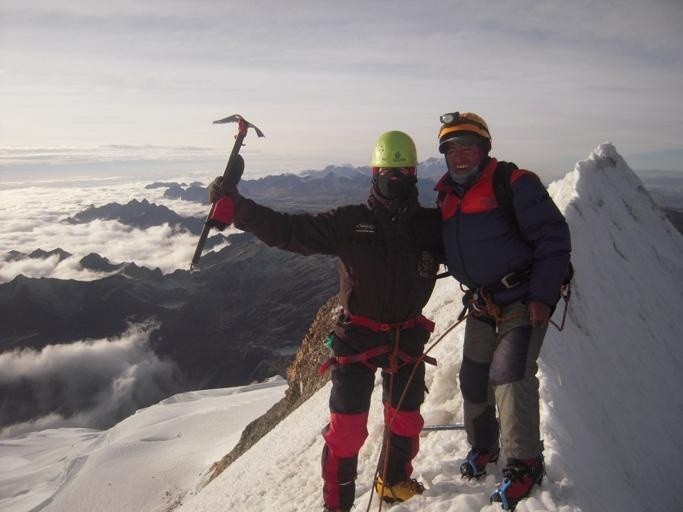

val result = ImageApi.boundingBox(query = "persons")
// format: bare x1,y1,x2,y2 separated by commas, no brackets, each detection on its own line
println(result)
427,107,574,510
205,129,451,512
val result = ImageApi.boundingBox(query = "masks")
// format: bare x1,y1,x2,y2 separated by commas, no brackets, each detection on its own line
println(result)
372,170,418,200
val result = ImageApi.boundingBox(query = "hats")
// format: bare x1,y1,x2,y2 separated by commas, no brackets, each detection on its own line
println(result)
438,134,482,154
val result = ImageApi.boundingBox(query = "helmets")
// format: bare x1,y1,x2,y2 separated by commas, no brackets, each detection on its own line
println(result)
437,111,492,151
371,131,418,169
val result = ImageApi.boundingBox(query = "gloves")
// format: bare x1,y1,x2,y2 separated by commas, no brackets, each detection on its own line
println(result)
208,176,255,229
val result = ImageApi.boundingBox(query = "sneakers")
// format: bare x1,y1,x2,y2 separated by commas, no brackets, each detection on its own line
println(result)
372,473,425,501
463,443,499,475
498,454,542,500
